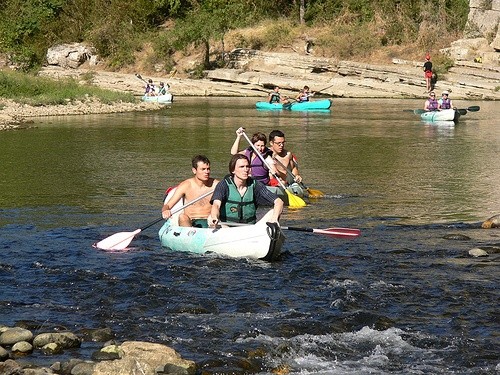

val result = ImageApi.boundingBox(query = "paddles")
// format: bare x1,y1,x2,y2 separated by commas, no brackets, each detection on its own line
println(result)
215,222,363,240
413,108,467,115
402,106,480,113
157,69,177,94
282,85,333,107
135,73,157,93
242,131,307,208
273,156,324,198
90,190,216,250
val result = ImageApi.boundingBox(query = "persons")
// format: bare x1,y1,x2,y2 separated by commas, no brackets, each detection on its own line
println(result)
161,155,221,228
145,79,155,96
207,154,284,227
423,55,432,92
268,86,287,104
294,86,315,103
437,93,453,110
424,92,438,111
231,127,286,187
267,130,302,187
158,82,170,95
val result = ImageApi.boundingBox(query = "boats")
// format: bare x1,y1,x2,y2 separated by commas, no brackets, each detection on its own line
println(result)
265,183,304,205
420,107,461,122
158,186,287,263
255,98,333,111
141,94,173,104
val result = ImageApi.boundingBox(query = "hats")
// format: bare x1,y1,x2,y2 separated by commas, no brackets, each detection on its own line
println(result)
425,55,430,59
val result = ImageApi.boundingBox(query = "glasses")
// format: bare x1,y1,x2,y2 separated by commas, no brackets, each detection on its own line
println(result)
272,139,286,144
431,94,435,96
